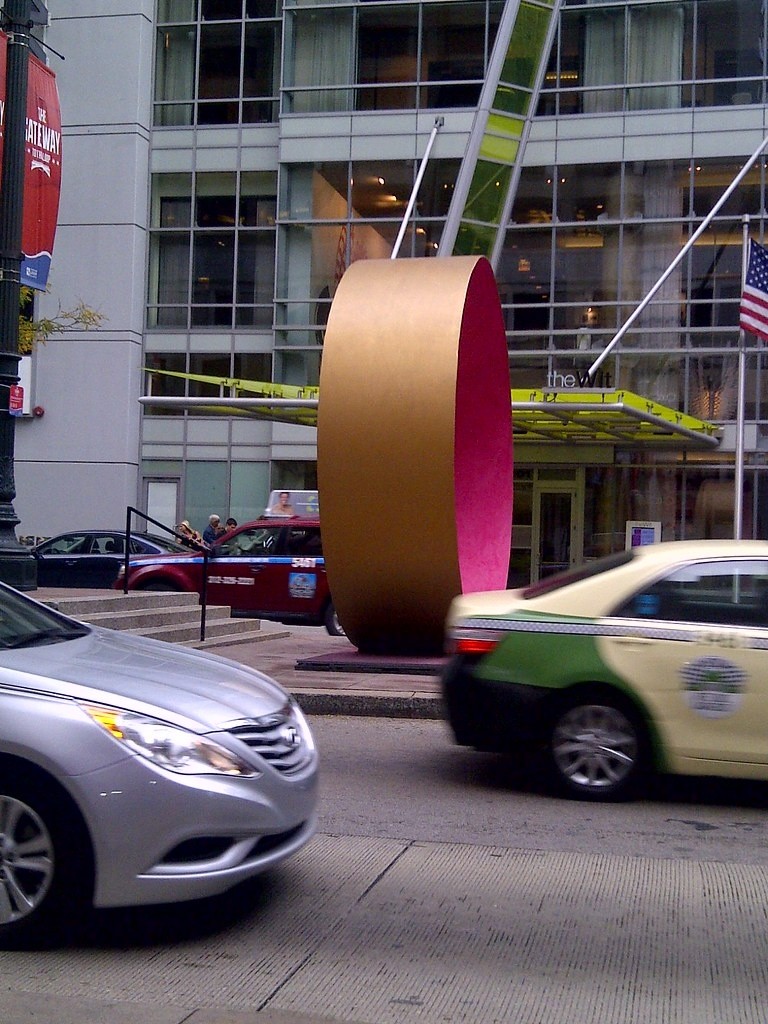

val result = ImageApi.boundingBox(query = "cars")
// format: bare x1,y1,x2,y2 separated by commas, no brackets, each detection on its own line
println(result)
439,536,768,802
29,529,173,588
0,579,320,949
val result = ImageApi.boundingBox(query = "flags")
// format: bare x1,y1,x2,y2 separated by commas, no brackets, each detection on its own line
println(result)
740,237,768,339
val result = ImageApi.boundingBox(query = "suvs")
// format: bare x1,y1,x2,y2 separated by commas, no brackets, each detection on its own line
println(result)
114,519,348,636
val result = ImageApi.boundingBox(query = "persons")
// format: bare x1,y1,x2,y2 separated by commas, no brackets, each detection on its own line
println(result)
215,518,237,555
273,492,294,515
203,514,222,556
178,520,201,546
216,523,224,532
